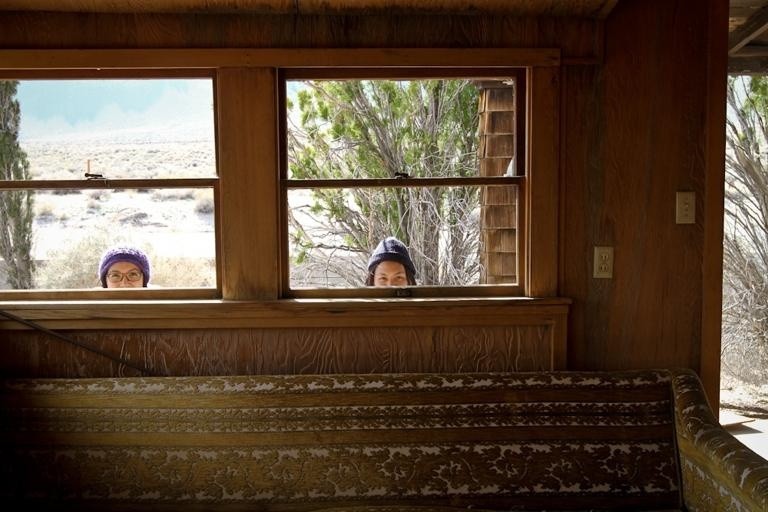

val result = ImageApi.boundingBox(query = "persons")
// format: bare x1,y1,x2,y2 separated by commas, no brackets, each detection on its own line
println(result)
98,249,150,288
363,236,416,287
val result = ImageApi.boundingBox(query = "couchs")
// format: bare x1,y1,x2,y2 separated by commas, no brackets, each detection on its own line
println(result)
0,368,768,512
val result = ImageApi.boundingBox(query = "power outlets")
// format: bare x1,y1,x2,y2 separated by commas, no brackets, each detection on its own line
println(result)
593,246,615,278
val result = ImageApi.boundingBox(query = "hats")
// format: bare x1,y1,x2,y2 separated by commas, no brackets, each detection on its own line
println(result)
367,237,417,274
99,243,151,286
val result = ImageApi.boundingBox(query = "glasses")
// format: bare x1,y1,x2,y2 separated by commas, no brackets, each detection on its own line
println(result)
105,268,143,283
